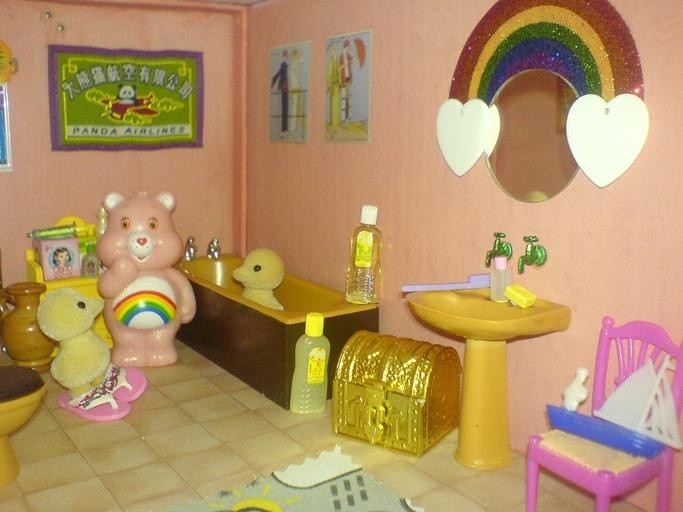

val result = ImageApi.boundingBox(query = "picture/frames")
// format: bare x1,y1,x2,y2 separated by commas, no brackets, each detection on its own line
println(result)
267,40,312,145
322,27,375,142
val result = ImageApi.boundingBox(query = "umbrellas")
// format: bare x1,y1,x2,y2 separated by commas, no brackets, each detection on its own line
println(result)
353,38,366,71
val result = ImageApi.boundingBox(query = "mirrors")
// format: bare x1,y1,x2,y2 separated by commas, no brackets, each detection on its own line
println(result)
482,68,578,205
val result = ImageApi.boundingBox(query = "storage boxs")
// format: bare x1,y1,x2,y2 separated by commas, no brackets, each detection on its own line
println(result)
328,330,461,458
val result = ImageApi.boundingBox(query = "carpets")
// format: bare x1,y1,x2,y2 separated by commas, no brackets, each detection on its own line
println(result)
156,443,429,511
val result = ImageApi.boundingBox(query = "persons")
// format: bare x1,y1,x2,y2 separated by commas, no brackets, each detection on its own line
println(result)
339,39,352,125
288,49,305,132
326,46,346,137
271,49,290,136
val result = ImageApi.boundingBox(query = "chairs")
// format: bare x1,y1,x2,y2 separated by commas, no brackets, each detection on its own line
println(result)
523,315,683,512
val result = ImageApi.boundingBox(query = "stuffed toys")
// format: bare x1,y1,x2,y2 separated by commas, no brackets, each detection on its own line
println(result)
231,246,286,312
35,285,112,401
